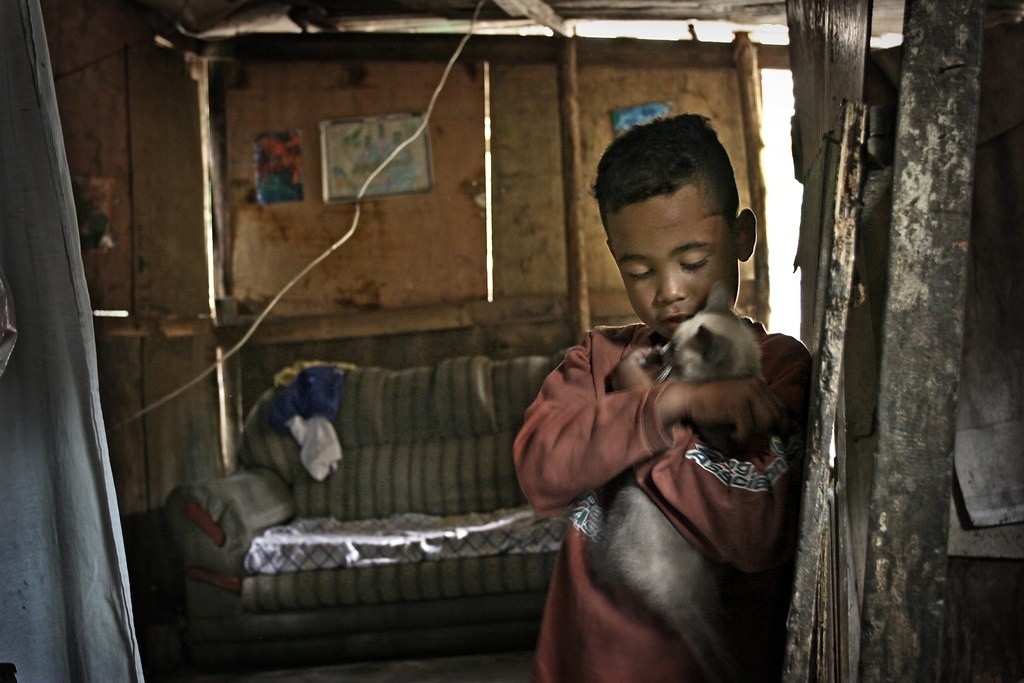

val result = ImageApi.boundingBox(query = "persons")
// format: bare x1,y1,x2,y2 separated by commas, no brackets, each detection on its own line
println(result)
513,113,812,683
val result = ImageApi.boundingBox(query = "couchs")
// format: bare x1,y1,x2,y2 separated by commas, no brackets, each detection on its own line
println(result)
168,351,583,673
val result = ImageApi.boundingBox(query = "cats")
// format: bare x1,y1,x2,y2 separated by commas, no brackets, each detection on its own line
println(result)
590,281,763,683
